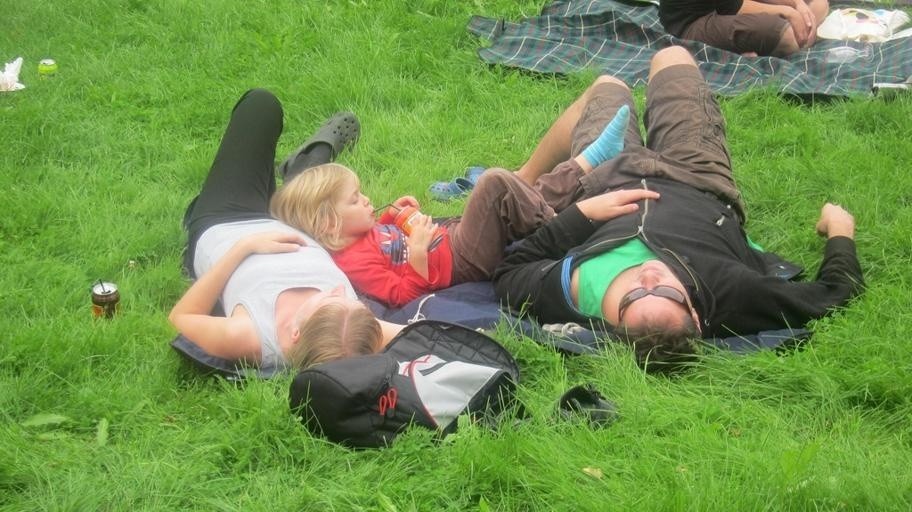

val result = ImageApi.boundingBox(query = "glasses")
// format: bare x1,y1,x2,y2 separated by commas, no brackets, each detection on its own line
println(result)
616,285,693,321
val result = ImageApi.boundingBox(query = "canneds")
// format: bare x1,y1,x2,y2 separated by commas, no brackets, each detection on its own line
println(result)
393,204,443,252
37,58,58,77
92,281,121,320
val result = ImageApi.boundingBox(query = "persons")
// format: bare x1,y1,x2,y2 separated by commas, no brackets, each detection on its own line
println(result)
166,84,409,374
491,43,864,378
269,104,632,312
658,0,830,60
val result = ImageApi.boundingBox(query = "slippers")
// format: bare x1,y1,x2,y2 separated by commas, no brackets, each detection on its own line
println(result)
278,113,360,181
427,165,485,202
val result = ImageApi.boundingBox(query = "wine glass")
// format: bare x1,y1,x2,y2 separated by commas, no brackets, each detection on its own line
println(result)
825,43,874,64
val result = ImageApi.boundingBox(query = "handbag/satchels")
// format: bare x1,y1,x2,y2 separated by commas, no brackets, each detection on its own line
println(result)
289,321,520,454
